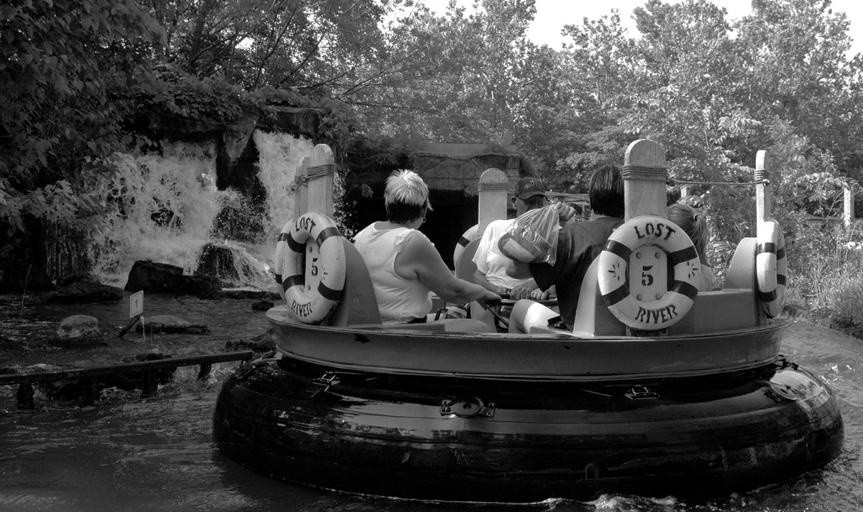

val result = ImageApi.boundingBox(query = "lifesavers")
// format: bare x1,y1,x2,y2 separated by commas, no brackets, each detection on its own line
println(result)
275,218,292,303
594,215,701,331
756,217,789,321
280,211,348,325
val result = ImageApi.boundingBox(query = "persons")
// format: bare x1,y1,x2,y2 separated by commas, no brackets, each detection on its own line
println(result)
496,162,624,332
471,172,577,303
341,168,503,331
666,203,722,290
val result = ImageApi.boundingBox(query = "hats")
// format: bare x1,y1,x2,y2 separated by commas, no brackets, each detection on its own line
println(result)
514,176,550,201
